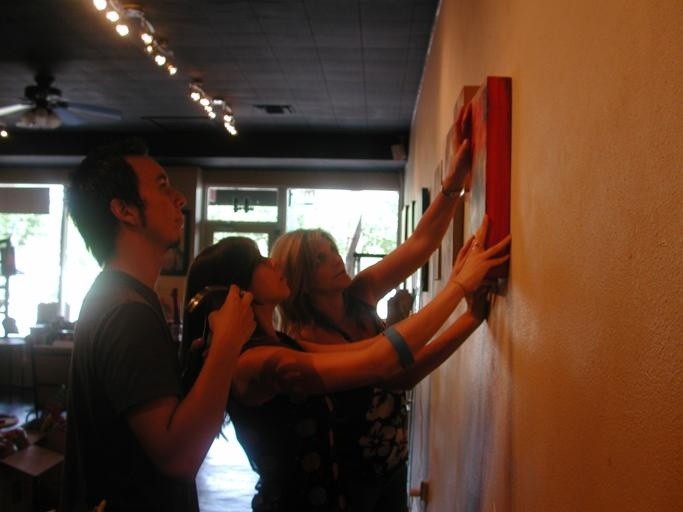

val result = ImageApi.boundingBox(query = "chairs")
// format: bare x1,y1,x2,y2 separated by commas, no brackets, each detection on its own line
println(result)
0,338,72,420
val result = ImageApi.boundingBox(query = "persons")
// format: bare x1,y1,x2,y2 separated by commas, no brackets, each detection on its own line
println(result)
66,142,256,510
180,101,513,511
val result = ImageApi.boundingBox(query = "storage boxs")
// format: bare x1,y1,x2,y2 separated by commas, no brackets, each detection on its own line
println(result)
0,430,75,509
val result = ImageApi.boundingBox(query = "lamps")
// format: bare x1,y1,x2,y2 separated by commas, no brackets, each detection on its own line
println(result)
138,27,181,76
17,103,63,130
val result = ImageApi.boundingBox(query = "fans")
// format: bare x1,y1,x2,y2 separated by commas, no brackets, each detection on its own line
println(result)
0,71,123,127
